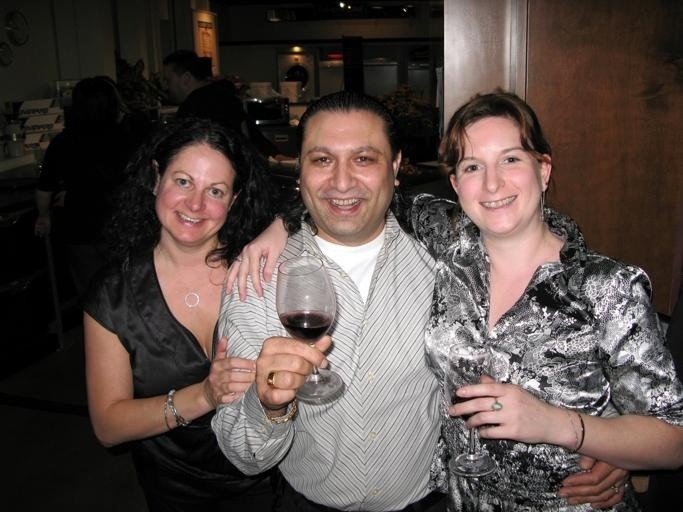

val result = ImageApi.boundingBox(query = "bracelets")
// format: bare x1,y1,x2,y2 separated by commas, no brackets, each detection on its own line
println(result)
167,387,192,428
569,411,585,454
267,401,297,425
164,394,173,431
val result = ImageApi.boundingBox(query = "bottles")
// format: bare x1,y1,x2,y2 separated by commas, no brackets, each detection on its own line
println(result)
4,124,25,157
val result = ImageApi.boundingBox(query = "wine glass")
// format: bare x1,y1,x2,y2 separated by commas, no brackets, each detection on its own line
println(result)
445,344,497,478
277,256,344,405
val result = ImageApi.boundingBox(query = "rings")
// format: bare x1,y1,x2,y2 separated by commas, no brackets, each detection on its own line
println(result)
610,485,619,494
267,371,276,388
492,396,502,412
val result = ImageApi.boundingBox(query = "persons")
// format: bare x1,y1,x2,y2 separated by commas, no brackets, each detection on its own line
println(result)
162,49,244,135
79,111,288,512
33,75,138,297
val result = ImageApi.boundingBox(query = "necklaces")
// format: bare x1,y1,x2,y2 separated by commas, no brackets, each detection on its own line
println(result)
156,244,217,309
